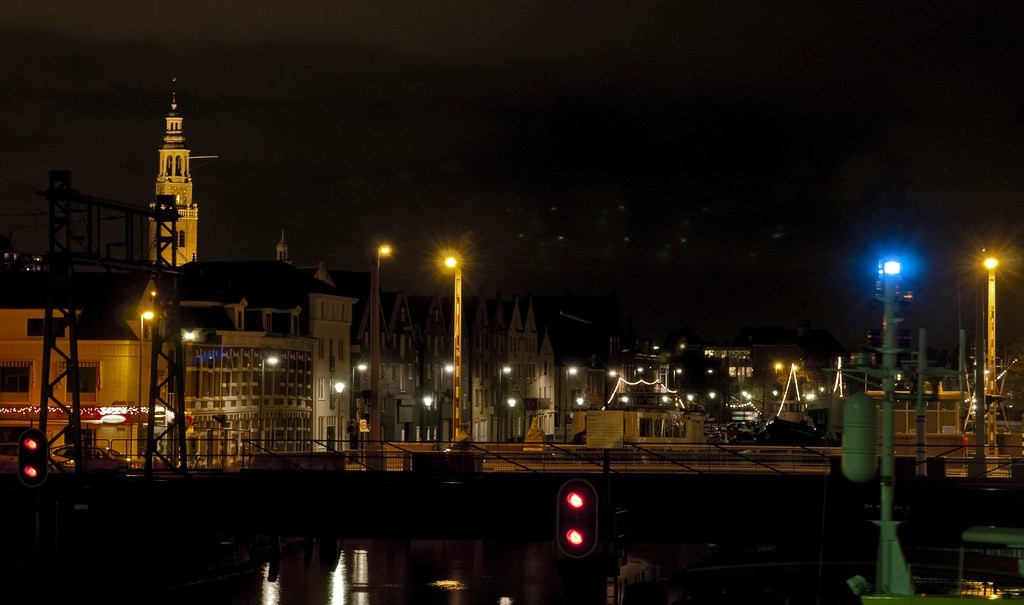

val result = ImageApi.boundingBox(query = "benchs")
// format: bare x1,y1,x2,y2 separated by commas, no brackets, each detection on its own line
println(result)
962,525,1024,577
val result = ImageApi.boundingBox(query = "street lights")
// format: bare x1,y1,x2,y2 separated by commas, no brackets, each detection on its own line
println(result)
333,381,345,451
440,252,466,450
709,392,716,431
350,363,367,420
136,310,155,458
370,244,393,449
564,366,578,443
258,357,279,452
496,365,513,443
507,398,516,440
984,257,998,455
576,397,584,412
687,394,694,409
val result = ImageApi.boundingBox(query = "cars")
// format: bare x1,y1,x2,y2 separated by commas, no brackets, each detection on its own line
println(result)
715,421,755,441
51,446,129,474
0,442,21,475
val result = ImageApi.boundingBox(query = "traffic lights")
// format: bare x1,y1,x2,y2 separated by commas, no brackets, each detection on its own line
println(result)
18,429,49,489
557,478,599,558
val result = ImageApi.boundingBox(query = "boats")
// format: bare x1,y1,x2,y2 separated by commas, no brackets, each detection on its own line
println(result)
822,256,1024,605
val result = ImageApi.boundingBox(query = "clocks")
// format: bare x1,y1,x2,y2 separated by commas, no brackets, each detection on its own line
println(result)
169,185,187,205
